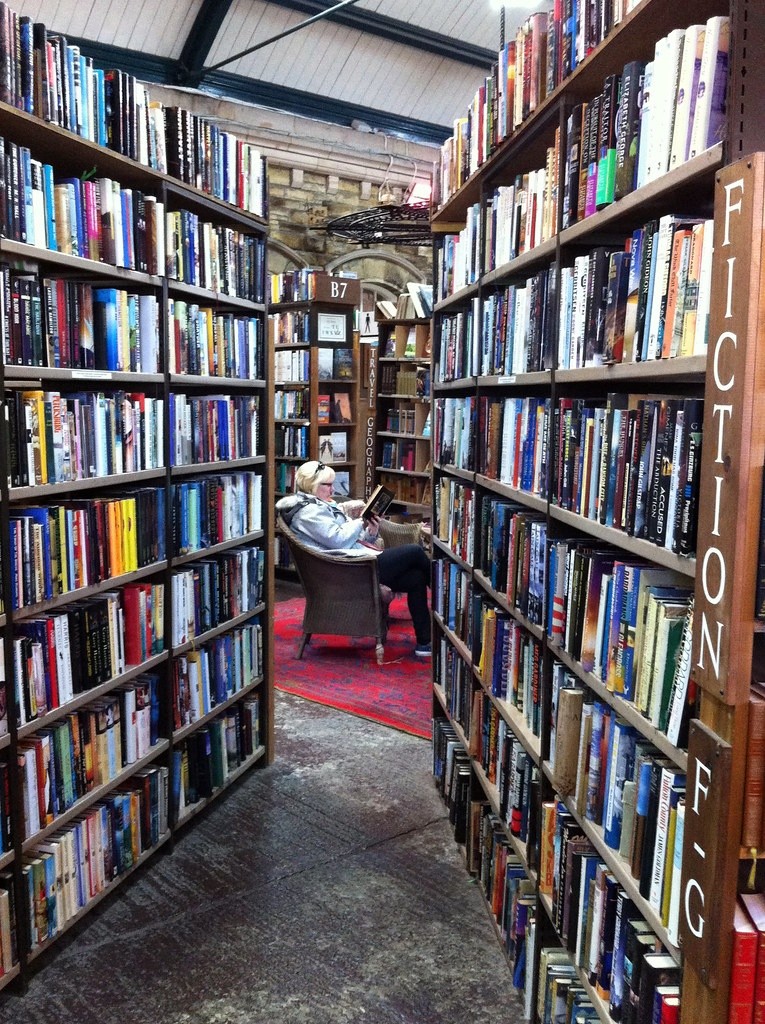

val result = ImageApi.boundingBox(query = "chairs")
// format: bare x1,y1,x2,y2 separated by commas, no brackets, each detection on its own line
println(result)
277,494,426,663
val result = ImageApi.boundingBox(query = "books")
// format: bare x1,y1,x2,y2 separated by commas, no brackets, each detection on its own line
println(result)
0,1,274,978
263,267,432,572
431,0,765,1024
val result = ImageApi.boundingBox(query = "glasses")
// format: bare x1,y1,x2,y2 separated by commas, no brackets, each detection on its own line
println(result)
322,482,334,488
314,461,325,474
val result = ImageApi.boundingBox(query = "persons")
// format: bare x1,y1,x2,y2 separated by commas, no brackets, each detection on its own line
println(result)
275,460,431,657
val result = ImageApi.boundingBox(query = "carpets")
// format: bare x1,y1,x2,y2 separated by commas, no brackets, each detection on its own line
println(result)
273,588,434,740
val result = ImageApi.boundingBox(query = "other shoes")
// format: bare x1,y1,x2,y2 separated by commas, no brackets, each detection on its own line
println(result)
415,642,431,657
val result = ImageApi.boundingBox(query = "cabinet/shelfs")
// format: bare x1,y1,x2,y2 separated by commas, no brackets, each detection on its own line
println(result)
274,267,362,590
431,0,765,1024
0,0,272,989
364,303,433,591
